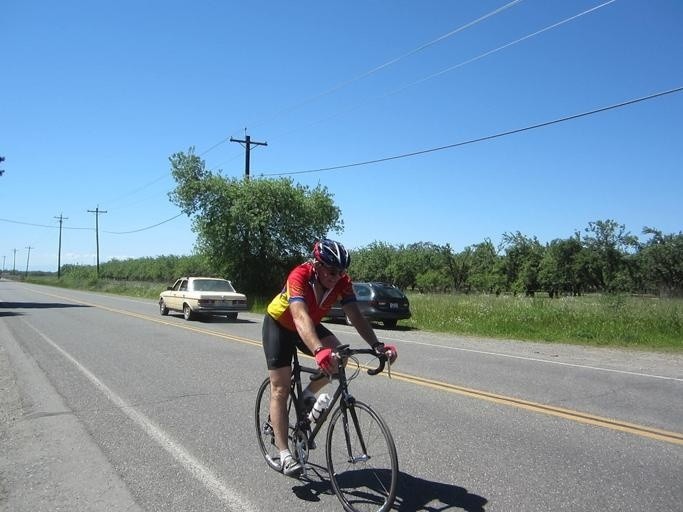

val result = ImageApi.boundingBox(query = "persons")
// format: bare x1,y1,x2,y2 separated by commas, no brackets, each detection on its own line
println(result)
260,239,398,477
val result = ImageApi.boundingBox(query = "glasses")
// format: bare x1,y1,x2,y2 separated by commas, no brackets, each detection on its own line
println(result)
322,264,346,277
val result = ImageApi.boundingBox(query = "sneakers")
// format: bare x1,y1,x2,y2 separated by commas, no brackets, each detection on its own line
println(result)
281,455,302,475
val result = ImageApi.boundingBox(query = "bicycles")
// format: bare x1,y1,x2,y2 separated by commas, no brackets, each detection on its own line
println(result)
255,334,398,512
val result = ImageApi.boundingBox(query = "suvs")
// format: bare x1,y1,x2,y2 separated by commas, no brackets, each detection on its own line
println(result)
321,282,413,329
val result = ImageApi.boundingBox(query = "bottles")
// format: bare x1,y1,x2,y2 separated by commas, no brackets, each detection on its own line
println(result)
307,392,330,422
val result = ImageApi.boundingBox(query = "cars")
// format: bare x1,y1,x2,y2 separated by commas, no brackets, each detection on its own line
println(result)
156,276,248,323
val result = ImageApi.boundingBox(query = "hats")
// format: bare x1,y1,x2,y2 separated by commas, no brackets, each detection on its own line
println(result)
315,240,351,268
